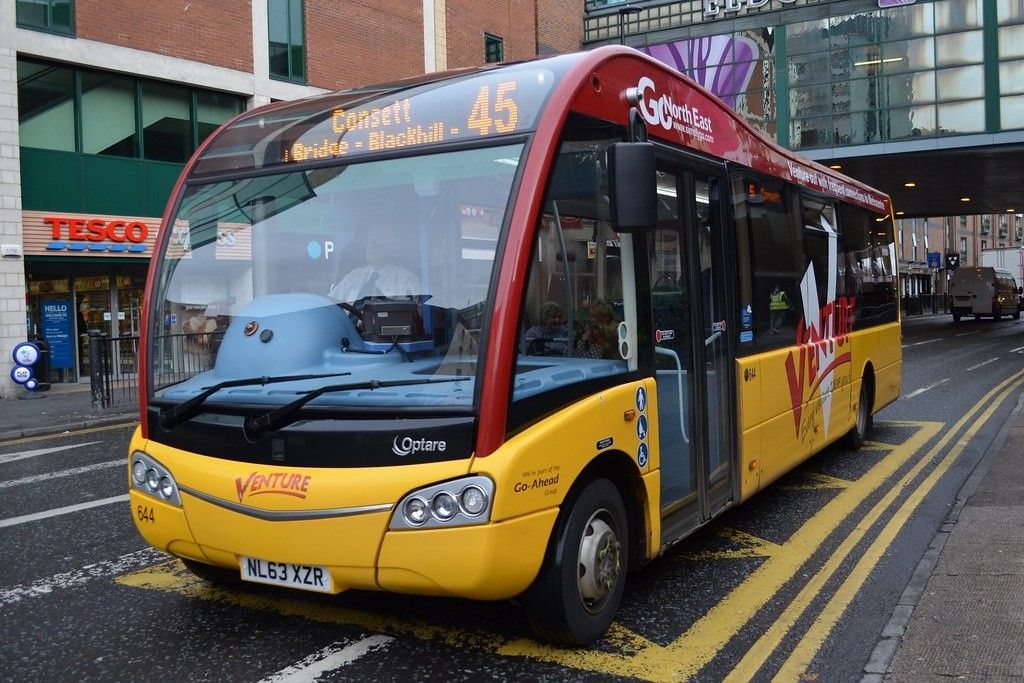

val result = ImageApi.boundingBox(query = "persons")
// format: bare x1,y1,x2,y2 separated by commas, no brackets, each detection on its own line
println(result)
577,301,620,359
325,226,422,316
524,301,572,355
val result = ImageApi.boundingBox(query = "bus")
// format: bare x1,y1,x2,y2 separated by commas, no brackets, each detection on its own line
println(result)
126,42,902,649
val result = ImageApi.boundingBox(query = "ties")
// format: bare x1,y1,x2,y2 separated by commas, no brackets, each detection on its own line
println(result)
347,272,380,329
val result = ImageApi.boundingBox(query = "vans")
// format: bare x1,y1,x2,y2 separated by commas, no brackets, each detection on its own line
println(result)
951,266,1024,321
